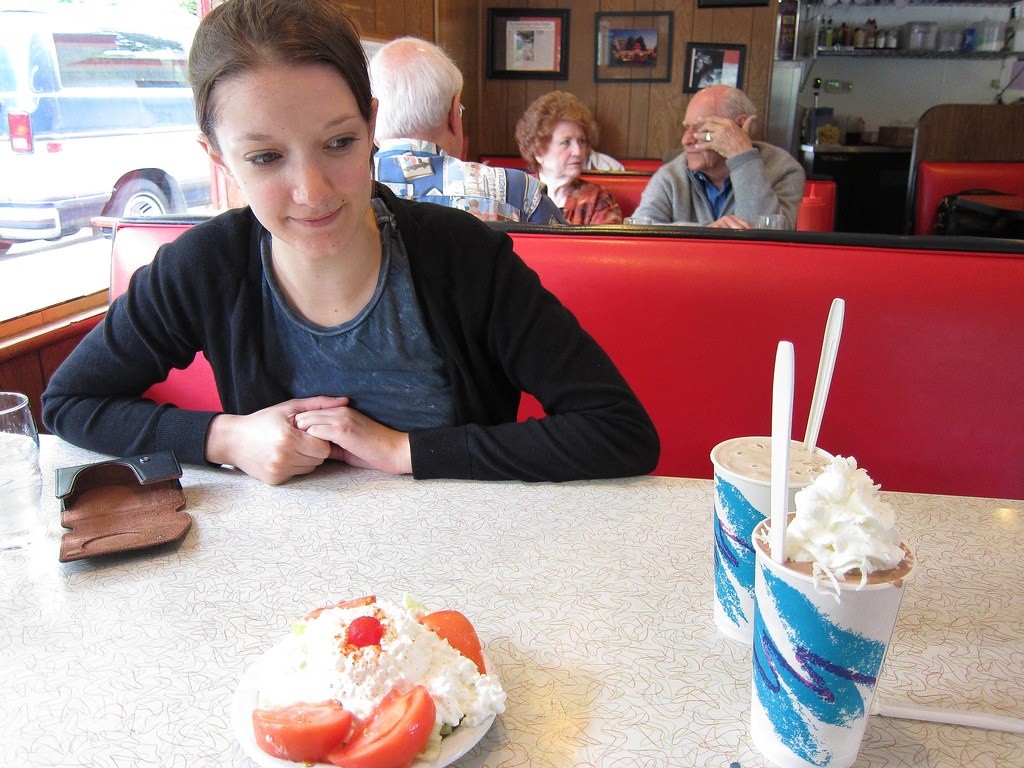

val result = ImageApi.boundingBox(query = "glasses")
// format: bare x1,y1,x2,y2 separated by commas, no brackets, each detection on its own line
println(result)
459,103,465,118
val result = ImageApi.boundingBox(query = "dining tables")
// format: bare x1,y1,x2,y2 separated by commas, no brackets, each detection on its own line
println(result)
0,431,1024,768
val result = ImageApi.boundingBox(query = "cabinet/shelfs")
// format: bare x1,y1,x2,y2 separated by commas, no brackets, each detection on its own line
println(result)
794,0,1024,180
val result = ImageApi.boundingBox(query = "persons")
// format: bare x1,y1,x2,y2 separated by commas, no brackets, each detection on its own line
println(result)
514,90,623,225
628,84,804,230
41,0,662,488
583,145,625,171
366,33,571,227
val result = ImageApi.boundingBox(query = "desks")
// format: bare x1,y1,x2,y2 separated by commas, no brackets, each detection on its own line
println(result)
954,194,1024,219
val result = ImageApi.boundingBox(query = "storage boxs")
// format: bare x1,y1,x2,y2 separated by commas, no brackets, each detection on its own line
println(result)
897,20,939,49
878,125,914,146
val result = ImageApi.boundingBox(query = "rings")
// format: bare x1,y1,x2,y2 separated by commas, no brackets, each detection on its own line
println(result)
705,132,712,142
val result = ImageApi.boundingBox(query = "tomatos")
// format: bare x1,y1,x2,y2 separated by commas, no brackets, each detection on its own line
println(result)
420,610,486,674
252,699,352,761
302,595,377,619
327,686,436,768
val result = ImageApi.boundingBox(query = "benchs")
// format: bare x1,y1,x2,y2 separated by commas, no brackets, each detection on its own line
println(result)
475,153,840,232
108,216,1024,500
914,161,1024,238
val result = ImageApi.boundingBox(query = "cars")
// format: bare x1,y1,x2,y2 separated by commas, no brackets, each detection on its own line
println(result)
1,0,226,253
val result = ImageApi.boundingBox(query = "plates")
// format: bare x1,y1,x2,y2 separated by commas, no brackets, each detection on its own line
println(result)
230,632,497,768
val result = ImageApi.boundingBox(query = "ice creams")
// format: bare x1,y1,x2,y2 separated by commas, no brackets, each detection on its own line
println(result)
763,456,905,583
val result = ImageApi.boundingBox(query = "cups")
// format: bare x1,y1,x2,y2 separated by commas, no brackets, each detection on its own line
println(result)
622,217,652,225
751,511,917,768
0,390,48,553
756,214,785,230
709,435,838,647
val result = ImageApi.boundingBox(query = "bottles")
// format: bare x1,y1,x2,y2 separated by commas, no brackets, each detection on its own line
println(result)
797,183,826,232
1005,7,1019,50
818,17,898,48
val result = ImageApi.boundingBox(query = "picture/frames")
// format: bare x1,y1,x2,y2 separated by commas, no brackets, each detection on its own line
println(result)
359,36,390,63
682,42,746,94
593,11,673,83
485,8,570,81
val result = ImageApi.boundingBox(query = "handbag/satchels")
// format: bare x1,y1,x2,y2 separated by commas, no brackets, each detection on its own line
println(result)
931,188,1024,238
